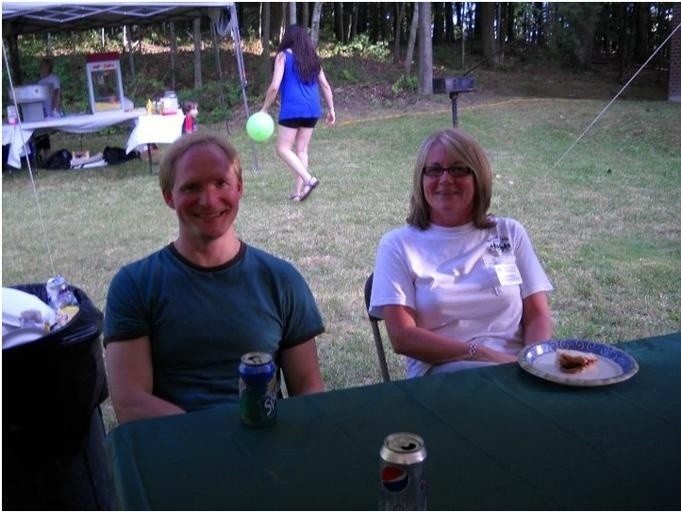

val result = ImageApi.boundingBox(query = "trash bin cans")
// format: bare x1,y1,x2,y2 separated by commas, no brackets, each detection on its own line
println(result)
2,282,107,512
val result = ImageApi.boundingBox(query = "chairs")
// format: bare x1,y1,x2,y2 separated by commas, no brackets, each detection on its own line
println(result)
364,272,392,383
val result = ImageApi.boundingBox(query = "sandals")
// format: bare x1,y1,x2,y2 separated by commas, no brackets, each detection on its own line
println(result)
300,176,319,200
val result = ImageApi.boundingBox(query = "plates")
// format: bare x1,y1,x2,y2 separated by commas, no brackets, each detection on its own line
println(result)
518,338,639,387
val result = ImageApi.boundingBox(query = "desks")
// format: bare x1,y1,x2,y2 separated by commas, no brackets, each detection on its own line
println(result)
1,108,184,175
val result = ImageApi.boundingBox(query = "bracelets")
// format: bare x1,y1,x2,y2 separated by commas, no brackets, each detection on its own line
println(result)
465,341,480,363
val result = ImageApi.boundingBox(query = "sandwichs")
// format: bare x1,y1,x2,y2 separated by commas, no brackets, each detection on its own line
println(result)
556,348,597,374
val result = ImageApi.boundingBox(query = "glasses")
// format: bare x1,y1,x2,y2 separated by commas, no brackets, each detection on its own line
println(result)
422,164,471,178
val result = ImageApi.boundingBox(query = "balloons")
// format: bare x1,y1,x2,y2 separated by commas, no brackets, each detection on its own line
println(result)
245,111,275,143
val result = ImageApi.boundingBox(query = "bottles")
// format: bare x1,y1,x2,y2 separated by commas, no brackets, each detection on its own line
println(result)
379,432,428,512
239,352,278,429
46,277,67,310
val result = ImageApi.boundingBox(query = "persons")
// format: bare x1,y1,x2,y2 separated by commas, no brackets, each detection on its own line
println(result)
37,59,61,165
260,23,336,205
368,128,554,381
101,131,326,425
180,100,200,137
